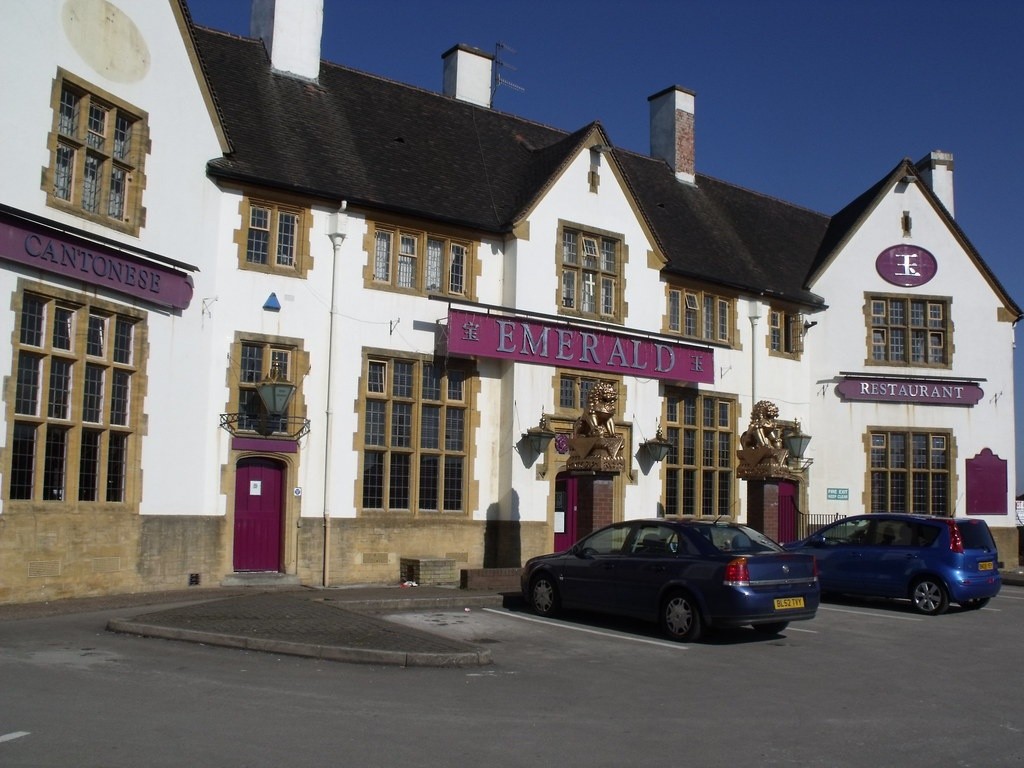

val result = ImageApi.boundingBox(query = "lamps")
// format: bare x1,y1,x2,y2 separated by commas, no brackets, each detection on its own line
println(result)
527,412,558,455
639,426,674,464
785,418,812,462
255,351,299,416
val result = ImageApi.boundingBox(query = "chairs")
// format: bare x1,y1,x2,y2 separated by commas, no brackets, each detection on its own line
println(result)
897,526,913,547
635,533,667,554
881,527,897,546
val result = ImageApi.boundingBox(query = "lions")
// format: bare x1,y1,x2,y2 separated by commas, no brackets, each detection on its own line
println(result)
740,400,782,448
571,382,618,439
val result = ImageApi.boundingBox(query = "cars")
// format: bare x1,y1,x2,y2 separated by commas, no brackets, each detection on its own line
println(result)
519,516,819,643
779,511,1004,616
